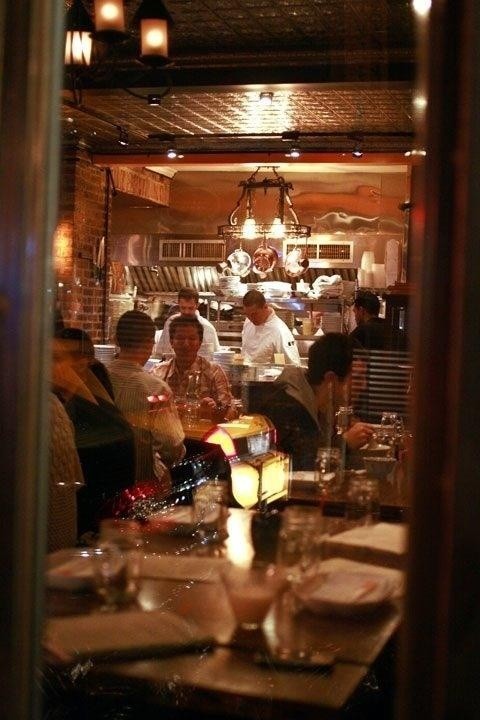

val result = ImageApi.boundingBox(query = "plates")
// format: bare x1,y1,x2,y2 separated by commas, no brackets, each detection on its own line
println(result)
148,504,222,539
313,280,356,301
298,566,397,616
359,444,392,457
213,349,234,380
93,345,116,364
44,548,128,592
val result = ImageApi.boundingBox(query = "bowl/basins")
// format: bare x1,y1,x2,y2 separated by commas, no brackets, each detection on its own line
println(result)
359,250,385,292
213,276,292,300
321,315,340,334
364,456,397,475
274,308,294,335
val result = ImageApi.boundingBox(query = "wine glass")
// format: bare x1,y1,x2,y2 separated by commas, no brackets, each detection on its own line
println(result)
190,478,228,560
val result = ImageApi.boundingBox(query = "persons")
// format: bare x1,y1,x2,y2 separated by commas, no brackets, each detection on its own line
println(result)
48,287,299,536
255,332,374,472
348,292,407,351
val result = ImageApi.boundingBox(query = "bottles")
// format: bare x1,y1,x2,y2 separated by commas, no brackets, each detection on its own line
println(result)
333,410,350,444
183,375,201,430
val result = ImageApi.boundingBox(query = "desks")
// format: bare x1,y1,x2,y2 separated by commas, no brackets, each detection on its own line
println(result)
42,408,407,720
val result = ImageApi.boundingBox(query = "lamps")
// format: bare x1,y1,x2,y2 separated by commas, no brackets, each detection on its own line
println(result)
218,164,312,278
63,1,171,74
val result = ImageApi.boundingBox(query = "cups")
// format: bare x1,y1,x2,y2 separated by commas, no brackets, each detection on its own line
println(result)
217,560,285,631
92,547,123,618
345,476,380,533
378,412,402,449
274,504,324,604
312,445,345,502
301,318,312,335
